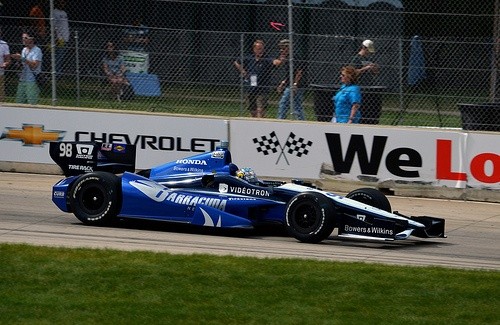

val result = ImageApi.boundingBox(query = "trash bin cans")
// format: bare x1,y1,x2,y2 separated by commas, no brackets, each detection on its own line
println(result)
457,103,500,131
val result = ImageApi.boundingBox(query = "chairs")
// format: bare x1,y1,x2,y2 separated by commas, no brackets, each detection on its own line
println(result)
229,163,263,187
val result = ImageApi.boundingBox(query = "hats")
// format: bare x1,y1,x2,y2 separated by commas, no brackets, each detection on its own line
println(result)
278,39,291,46
363,40,374,52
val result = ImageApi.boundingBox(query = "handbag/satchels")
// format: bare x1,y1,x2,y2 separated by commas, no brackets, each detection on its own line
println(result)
36,72,48,86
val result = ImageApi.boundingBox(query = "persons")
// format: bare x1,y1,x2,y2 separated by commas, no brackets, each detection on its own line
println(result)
234,40,384,124
236,167,258,185
0,0,151,105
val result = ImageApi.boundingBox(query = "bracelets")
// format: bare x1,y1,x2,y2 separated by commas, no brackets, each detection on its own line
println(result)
282,80,286,85
293,83,298,86
21,56,26,62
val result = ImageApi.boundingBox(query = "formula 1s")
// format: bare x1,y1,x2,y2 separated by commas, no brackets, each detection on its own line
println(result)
48,139,448,243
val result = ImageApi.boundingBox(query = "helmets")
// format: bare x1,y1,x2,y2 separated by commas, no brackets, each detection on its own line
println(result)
235,167,260,186
